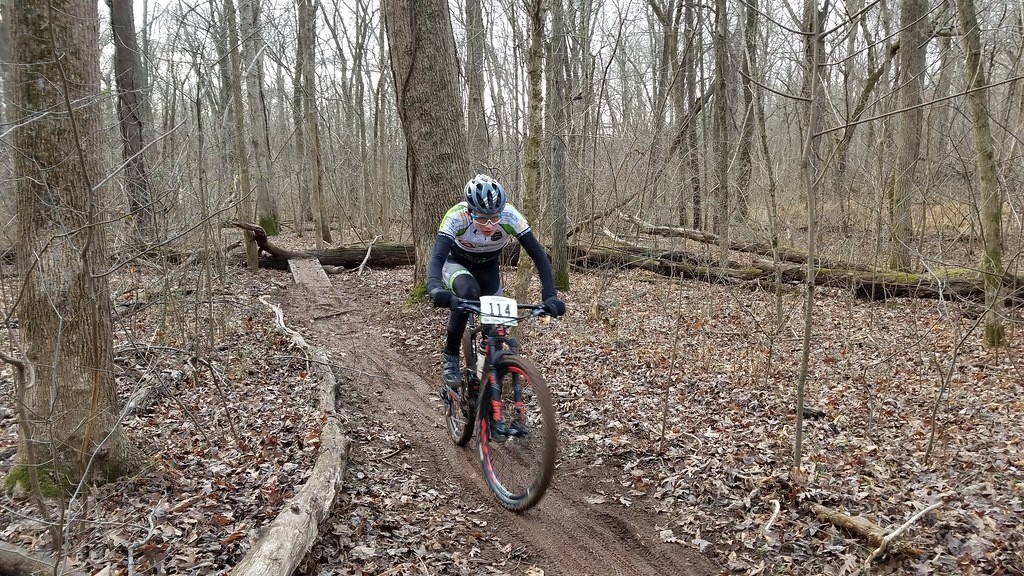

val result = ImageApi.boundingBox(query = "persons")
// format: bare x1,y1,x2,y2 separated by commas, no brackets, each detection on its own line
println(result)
426,174,566,442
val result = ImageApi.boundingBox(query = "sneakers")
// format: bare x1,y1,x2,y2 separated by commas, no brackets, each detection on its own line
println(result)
439,354,464,388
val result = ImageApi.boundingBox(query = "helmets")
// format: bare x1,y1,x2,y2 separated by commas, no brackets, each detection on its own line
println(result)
464,174,505,215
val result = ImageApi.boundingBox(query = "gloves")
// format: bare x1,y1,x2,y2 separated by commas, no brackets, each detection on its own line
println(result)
429,288,452,307
545,297,566,317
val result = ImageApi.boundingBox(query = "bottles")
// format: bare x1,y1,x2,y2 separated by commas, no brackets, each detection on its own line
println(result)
476,338,488,379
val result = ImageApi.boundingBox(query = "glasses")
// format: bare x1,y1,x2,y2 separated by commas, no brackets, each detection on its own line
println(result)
470,215,503,225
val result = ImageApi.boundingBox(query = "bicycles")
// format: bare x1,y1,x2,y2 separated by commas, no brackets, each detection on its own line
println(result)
433,294,560,513
